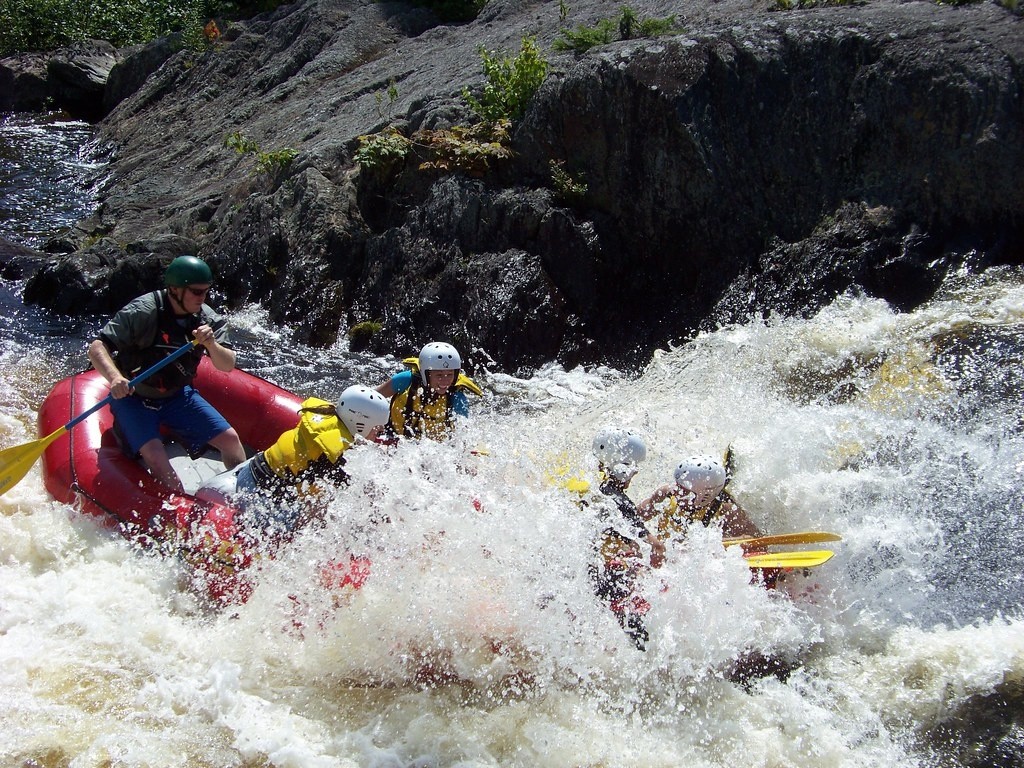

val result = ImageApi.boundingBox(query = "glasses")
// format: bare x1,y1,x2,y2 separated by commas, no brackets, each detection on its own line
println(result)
187,286,212,296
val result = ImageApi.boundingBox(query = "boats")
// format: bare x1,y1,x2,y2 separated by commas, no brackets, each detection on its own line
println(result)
34,354,387,604
587,524,804,686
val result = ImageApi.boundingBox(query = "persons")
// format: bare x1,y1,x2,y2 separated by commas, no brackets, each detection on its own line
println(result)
88,255,247,493
236,340,471,532
578,427,768,595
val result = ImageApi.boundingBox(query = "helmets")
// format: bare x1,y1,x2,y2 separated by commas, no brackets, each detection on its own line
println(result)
592,426,647,468
335,384,390,441
675,454,727,508
418,341,461,387
165,255,212,288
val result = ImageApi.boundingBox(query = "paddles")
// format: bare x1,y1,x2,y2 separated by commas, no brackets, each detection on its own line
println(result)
0,339,199,497
740,549,835,569
723,531,844,549
